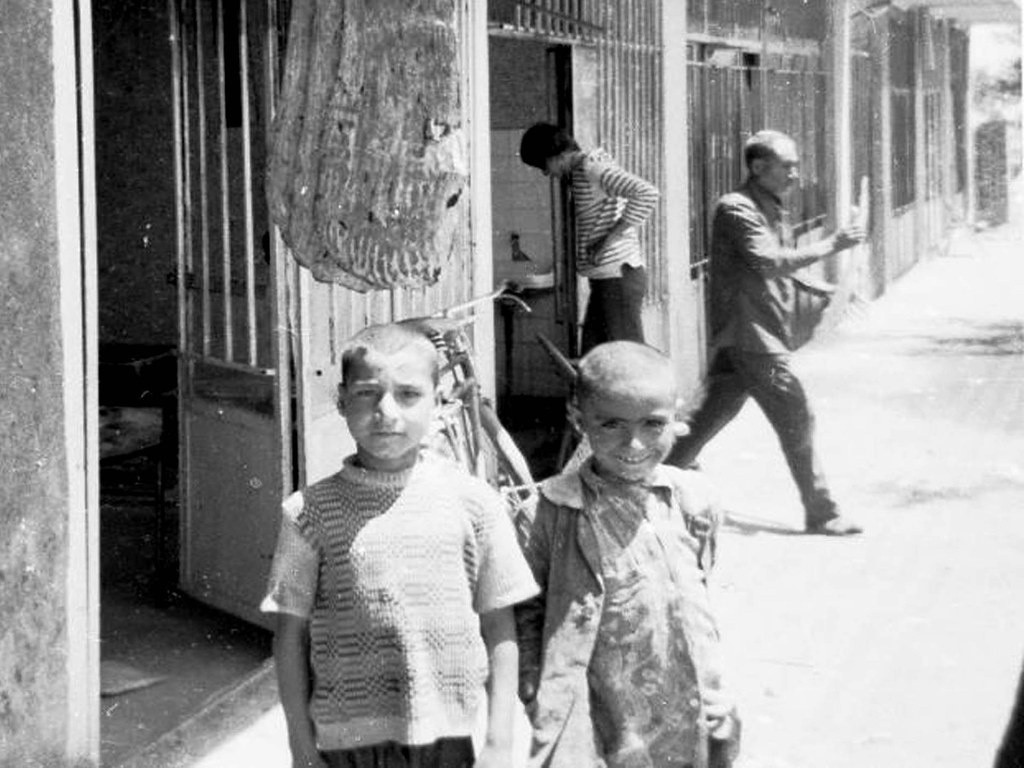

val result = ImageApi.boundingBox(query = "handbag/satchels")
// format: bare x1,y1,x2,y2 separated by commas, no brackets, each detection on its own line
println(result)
775,272,835,349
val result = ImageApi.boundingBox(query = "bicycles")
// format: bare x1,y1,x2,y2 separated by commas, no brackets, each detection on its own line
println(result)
397,279,540,519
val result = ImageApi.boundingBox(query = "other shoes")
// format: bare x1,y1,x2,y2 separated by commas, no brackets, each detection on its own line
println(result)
808,516,864,535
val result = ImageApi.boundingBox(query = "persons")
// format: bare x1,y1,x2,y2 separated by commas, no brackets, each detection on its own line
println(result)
260,324,541,768
664,128,868,536
511,339,740,768
518,122,658,470
